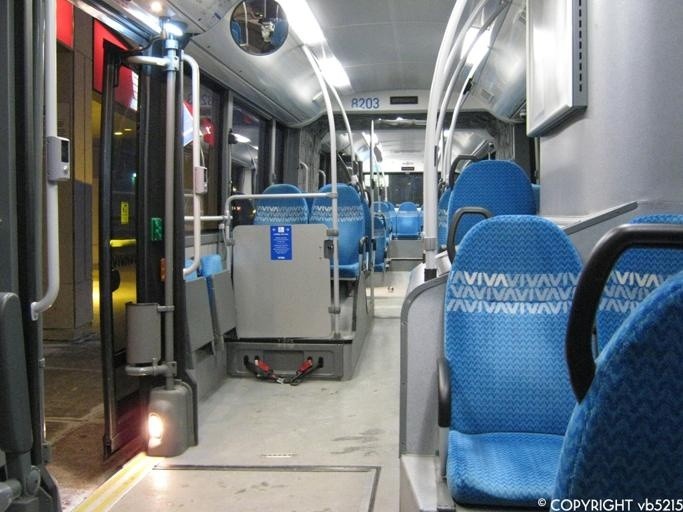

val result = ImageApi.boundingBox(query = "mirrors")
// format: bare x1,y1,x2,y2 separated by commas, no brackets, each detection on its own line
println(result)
229,0,290,57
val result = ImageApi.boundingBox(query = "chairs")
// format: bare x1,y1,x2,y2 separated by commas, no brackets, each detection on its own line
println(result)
266,18,287,50
240,158,682,512
230,21,242,45
